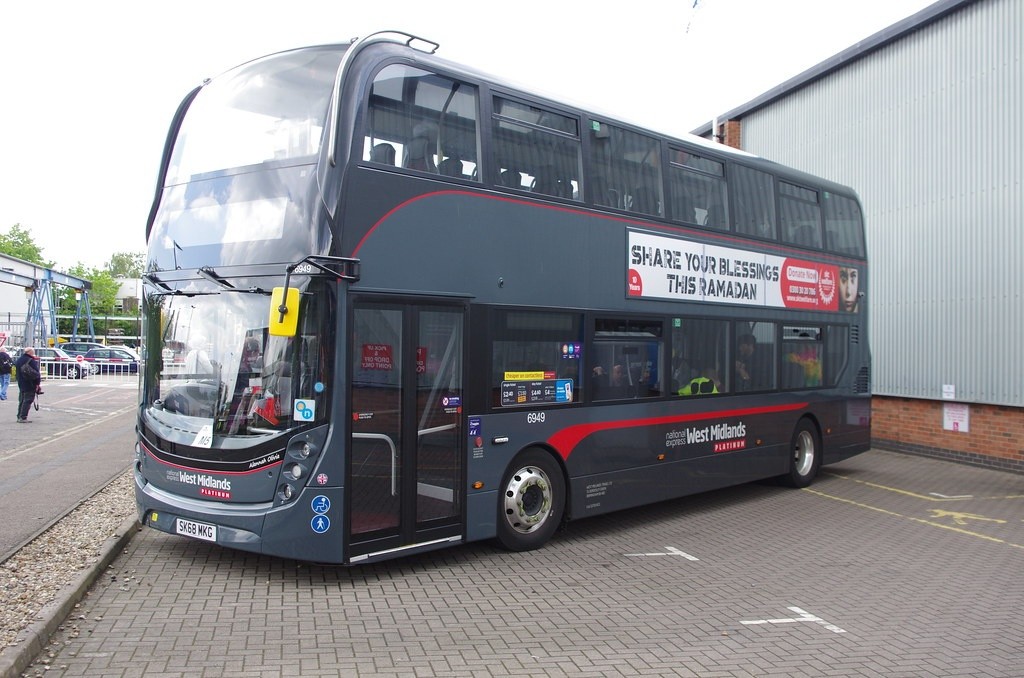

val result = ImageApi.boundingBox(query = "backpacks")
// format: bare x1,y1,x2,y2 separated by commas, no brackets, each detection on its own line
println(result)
1,353,12,373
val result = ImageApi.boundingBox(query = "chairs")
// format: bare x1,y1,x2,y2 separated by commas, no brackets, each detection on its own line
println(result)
367,135,840,253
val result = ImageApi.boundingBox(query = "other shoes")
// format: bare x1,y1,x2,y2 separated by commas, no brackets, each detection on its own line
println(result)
0,395,7,400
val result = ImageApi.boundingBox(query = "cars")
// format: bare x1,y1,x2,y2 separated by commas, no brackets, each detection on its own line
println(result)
58,342,140,372
10,347,89,379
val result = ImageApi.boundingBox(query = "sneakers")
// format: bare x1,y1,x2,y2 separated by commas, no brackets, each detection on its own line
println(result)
16,417,31,422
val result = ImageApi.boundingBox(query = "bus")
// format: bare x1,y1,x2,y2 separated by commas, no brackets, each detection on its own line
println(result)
130,27,872,567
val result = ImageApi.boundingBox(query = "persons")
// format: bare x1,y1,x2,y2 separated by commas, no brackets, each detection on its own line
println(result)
838,266,858,313
593,334,755,401
0,346,12,400
16,347,41,423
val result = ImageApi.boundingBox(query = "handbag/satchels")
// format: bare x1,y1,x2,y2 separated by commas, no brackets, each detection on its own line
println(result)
20,358,41,386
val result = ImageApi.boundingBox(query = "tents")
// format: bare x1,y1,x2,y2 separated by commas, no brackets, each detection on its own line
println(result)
48,337,68,348
